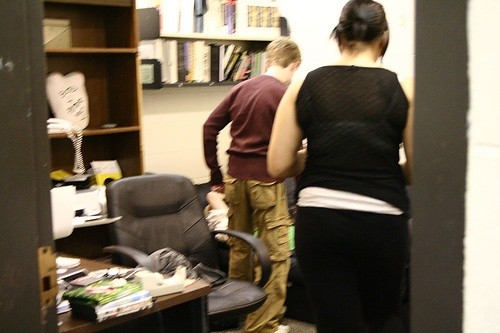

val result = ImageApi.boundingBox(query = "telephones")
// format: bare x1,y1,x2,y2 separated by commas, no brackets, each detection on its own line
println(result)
46,117,72,135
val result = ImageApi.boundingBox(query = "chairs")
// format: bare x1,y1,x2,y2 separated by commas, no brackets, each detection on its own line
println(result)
103,172,273,333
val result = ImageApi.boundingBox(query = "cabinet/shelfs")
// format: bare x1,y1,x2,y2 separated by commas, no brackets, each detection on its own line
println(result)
137,8,288,90
43,0,145,260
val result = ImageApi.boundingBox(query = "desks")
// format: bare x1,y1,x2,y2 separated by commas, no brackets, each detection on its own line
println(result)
56,251,211,333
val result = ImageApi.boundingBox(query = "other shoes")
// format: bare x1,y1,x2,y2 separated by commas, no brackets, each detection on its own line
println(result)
269,323,291,333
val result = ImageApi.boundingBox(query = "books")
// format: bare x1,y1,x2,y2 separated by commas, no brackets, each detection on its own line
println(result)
238,0,281,38
159,0,194,34
204,0,236,35
210,43,267,83
62,279,153,322
138,39,211,82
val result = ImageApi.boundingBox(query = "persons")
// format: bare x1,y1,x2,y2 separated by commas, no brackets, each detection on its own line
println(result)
203,38,303,333
268,0,413,333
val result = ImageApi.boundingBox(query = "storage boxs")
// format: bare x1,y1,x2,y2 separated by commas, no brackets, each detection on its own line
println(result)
43,18,72,49
86,159,122,185
